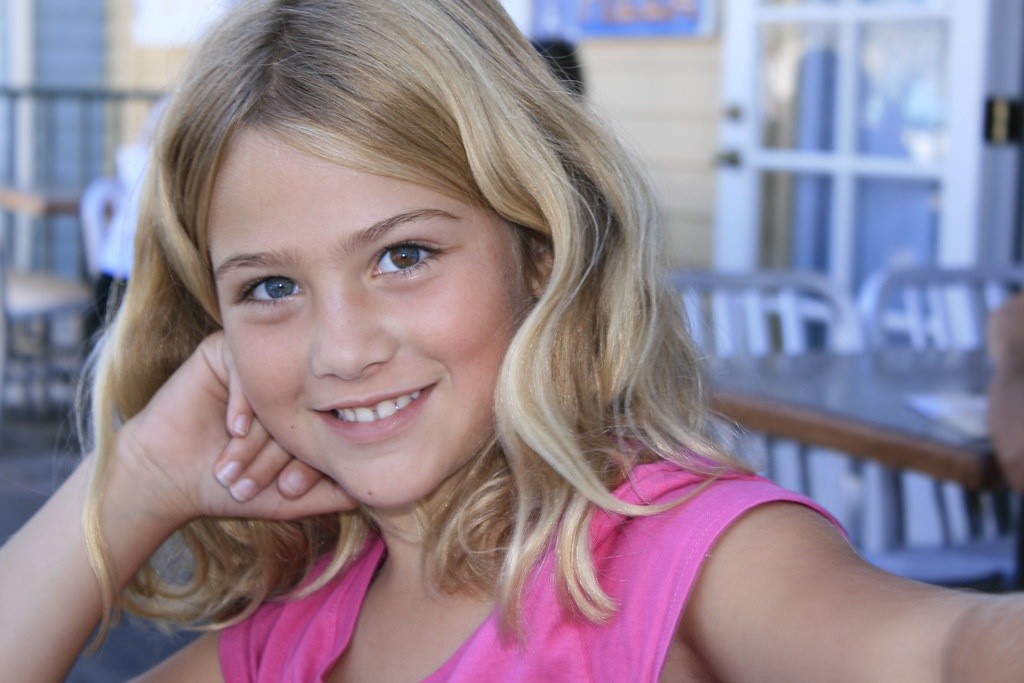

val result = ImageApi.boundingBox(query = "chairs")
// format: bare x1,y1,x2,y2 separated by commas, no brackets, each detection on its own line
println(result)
1,192,91,419
677,255,1024,595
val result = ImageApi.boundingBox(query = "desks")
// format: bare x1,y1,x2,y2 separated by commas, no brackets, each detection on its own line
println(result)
689,345,1002,491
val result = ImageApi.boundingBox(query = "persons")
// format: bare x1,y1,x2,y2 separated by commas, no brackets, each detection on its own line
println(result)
1,0,1024,683
